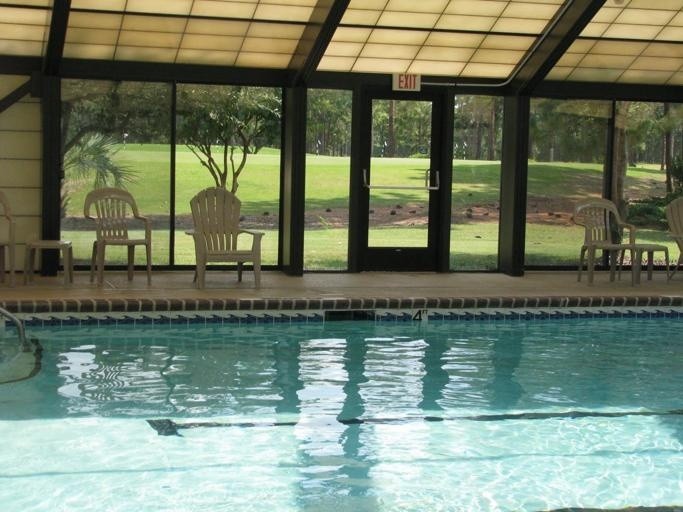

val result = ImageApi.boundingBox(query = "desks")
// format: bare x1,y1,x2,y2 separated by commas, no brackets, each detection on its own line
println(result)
23,240,74,286
619,244,670,284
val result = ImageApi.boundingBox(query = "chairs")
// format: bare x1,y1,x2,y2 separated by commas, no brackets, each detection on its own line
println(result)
84,187,152,287
0,189,15,287
572,197,636,288
185,187,265,289
665,197,683,282
